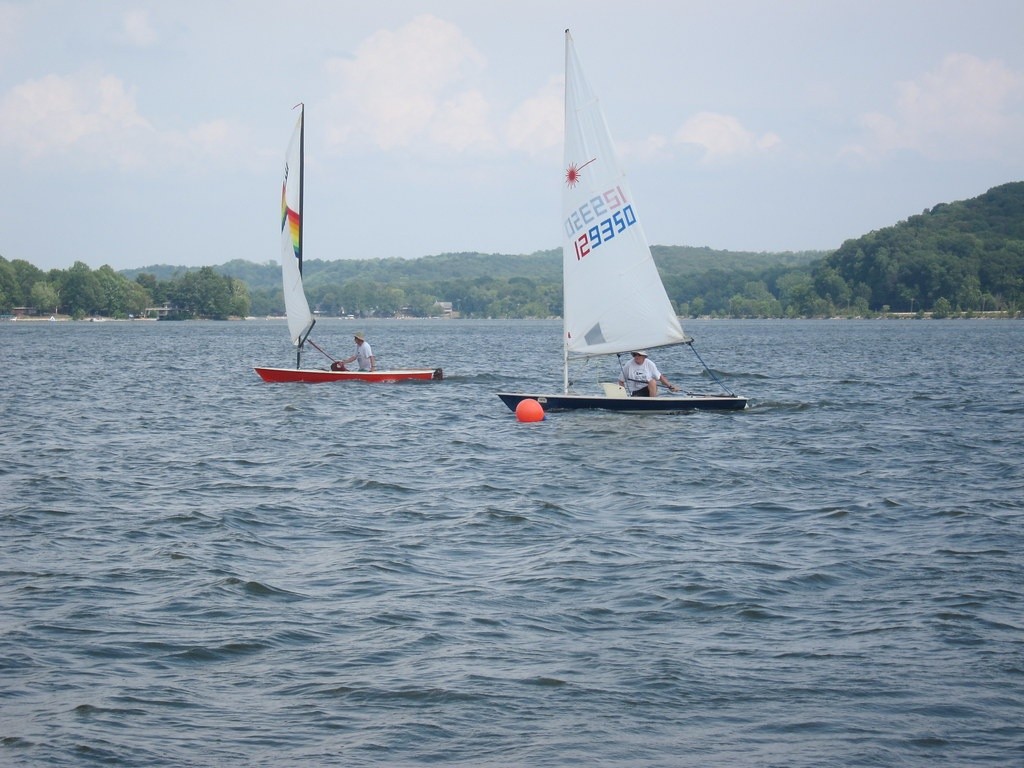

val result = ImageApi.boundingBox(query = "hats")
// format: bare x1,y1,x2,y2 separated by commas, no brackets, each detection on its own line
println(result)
353,331,364,342
631,349,649,358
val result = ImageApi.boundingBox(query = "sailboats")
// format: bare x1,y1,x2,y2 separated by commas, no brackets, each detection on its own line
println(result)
253,101,444,384
497,27,749,413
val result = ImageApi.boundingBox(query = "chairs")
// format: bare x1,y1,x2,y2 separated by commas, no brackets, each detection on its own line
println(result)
331,362,349,371
603,383,628,398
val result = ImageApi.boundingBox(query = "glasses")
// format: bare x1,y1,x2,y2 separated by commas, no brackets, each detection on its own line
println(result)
630,353,641,357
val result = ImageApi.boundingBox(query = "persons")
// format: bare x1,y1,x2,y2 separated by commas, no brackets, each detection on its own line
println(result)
343,332,375,372
618,350,677,397
